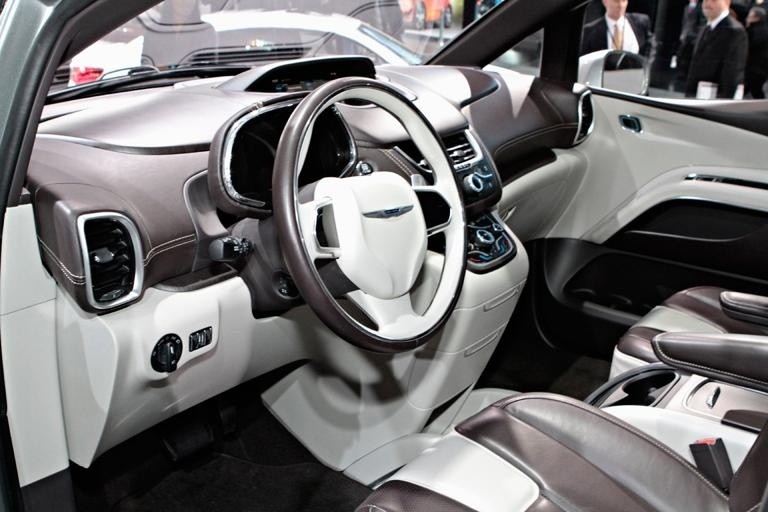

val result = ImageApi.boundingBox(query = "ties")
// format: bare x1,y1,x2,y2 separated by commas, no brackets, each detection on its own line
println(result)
612,24,623,49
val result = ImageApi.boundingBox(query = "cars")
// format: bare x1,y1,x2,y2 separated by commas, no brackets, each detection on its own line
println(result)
397,0,453,29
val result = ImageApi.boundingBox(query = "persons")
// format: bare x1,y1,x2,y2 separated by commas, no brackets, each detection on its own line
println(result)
141,0,220,65
582,0,651,95
653,1,768,100
